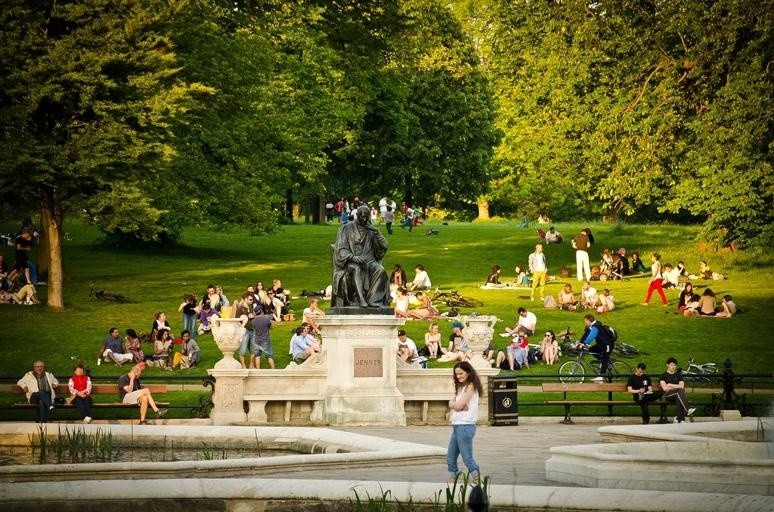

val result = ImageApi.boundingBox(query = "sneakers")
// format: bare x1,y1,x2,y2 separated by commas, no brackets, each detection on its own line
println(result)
139,422,152,425
83,416,94,422
640,301,650,306
156,410,168,416
661,303,669,308
429,355,438,359
687,409,697,416
590,375,605,381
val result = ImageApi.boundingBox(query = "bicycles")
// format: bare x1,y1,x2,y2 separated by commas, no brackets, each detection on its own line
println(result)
86,283,136,305
528,325,646,384
677,354,719,383
430,286,483,307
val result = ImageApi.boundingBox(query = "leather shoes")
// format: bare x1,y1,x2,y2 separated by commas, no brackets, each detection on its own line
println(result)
48,405,55,414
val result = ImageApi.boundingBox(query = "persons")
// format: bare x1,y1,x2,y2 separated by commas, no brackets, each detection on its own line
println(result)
331,207,393,308
659,357,696,423
325,197,424,235
396,321,470,369
178,283,230,337
574,315,615,384
66,364,92,423
446,362,483,493
235,278,331,369
389,263,433,320
97,312,200,371
119,366,168,423
485,214,736,318
0,224,41,305
17,360,59,424
482,307,558,372
627,362,664,422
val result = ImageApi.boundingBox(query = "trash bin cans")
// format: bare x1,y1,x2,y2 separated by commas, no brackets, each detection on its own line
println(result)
488,375,519,426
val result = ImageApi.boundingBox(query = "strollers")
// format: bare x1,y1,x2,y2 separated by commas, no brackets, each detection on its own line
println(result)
599,251,629,282
189,375,215,417
534,227,546,244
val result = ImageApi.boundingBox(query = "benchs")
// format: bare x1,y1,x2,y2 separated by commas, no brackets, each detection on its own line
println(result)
0,383,171,419
536,382,690,424
